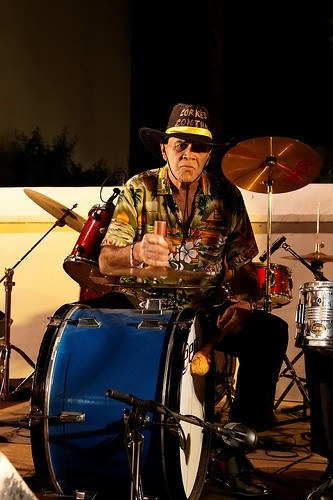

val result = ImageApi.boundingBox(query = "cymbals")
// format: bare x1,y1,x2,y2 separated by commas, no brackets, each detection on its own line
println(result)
99,265,218,290
221,136,322,194
24,189,87,233
280,252,333,264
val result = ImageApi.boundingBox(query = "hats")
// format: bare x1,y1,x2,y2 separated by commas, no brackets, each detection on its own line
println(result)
139,103,235,152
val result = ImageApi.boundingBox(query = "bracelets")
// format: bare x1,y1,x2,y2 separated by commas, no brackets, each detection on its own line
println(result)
243,298,256,309
130,243,142,267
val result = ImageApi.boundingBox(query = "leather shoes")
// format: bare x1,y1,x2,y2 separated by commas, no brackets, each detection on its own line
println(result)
204,453,270,498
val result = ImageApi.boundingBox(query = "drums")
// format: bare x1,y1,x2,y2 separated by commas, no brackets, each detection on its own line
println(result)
79,286,103,301
294,281,333,353
63,204,120,294
29,303,209,500
252,261,293,308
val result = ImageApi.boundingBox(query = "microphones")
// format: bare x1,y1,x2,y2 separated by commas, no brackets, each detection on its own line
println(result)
259,235,286,262
220,423,259,450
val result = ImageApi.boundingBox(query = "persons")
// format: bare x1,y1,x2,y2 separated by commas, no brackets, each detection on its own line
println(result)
99,103,289,431
